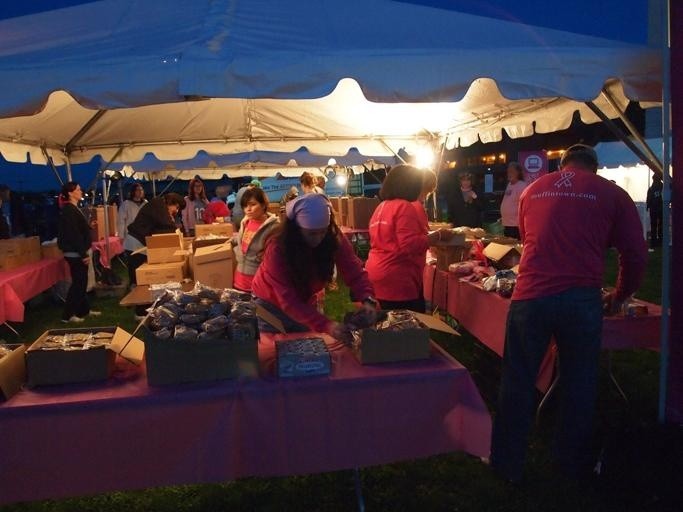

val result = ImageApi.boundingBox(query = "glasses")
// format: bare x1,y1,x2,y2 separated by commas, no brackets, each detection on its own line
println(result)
461,177,471,181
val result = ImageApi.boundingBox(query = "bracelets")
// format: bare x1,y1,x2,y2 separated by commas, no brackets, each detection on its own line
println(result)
436,230,442,242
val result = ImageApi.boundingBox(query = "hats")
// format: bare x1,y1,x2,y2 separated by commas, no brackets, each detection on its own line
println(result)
560,144,598,165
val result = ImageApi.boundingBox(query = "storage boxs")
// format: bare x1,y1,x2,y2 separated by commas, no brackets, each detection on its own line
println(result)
344,305,461,366
1,235,42,272
143,278,263,387
81,206,105,242
39,238,60,260
106,204,121,239
2,340,24,401
481,238,523,268
96,204,108,236
138,196,376,288
429,229,464,271
273,335,331,381
25,327,145,390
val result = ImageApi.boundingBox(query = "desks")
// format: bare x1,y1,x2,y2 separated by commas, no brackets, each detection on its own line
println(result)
0,328,494,512
427,234,672,409
0,253,71,324
91,236,131,278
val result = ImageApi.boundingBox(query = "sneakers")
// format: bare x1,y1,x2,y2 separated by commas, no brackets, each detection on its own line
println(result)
62,315,85,323
89,310,101,317
495,463,523,483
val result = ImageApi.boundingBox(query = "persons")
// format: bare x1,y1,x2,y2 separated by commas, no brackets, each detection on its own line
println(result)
231,186,282,294
363,164,456,315
499,160,529,240
479,142,647,479
11,188,58,241
278,185,299,206
299,169,323,197
59,181,102,324
180,176,211,237
646,173,663,253
251,193,381,342
1,183,11,237
447,172,484,229
411,172,437,231
209,178,233,202
316,177,325,188
1,191,26,339
122,190,188,322
117,181,149,254
230,179,263,232
